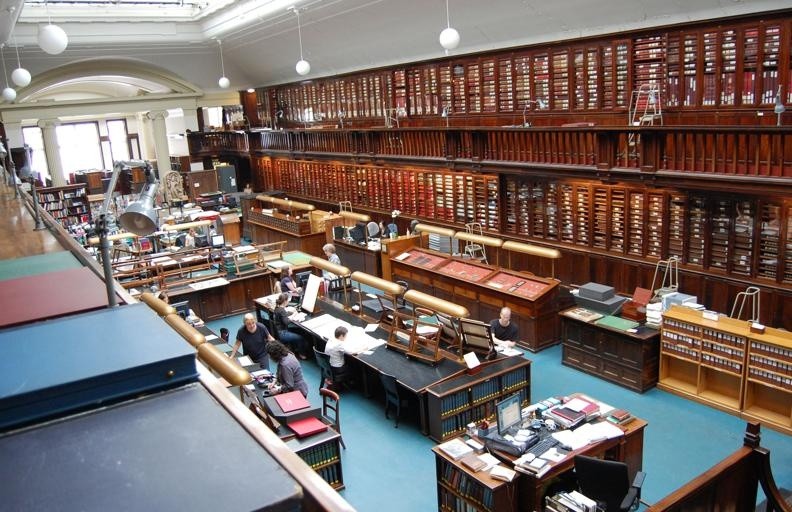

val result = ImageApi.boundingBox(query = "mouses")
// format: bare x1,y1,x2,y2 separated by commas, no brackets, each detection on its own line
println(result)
561,444,571,452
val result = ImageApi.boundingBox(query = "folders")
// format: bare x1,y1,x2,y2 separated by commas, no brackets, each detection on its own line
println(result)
545,489,596,511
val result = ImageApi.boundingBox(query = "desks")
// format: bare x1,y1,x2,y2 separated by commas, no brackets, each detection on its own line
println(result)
558,306,659,395
428,391,649,512
245,206,326,266
317,273,526,380
190,315,335,440
332,237,381,278
253,286,467,436
56,190,322,315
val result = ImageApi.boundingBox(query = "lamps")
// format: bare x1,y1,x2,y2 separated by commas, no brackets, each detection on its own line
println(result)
37,2,68,56
100,156,161,310
5,6,31,88
0,42,16,104
287,6,311,75
439,0,461,49
215,39,230,89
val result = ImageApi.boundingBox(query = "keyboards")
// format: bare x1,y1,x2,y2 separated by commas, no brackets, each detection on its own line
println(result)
525,435,559,456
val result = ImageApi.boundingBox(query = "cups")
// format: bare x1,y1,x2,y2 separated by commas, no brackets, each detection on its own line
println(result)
546,419,556,430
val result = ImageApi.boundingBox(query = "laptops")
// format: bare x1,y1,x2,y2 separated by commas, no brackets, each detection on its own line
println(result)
285,295,301,306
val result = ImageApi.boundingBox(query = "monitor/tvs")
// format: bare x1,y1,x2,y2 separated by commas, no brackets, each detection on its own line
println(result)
170,301,190,320
209,233,226,249
350,222,370,242
295,271,312,287
175,233,187,247
496,392,522,436
195,235,208,248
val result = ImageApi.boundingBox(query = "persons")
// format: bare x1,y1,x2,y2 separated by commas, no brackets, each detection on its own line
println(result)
268,339,307,400
185,228,200,248
273,293,308,361
377,220,390,238
243,183,254,196
324,326,359,386
321,243,340,285
229,312,275,372
488,306,516,348
279,265,297,292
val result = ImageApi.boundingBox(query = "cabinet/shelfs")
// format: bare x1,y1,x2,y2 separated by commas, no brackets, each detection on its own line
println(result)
426,357,533,443
237,7,791,185
283,429,346,493
391,246,572,353
172,156,190,175
189,151,792,329
35,182,94,231
656,304,751,423
742,328,791,435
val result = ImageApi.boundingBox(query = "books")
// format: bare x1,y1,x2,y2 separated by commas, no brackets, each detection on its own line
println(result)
438,485,479,512
48,209,92,219
512,452,553,478
438,365,528,415
441,459,492,510
219,245,260,273
544,489,597,512
442,385,529,440
428,233,459,252
542,393,635,427
296,440,340,469
34,186,86,202
551,422,624,449
170,301,205,328
57,215,91,228
40,202,89,211
316,464,341,485
437,436,515,482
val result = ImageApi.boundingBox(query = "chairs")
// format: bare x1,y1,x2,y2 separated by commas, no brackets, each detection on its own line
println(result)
311,387,347,452
572,455,649,512
387,224,397,238
220,327,231,340
365,222,378,237
268,320,297,356
379,371,409,428
313,345,357,401
